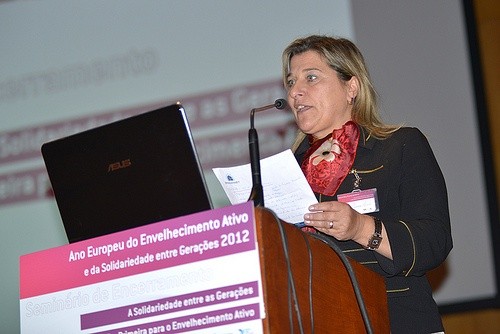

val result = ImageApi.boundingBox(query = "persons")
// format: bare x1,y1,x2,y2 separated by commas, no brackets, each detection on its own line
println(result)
281,36,453,334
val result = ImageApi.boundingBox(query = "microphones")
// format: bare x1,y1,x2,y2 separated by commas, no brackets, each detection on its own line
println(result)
246,99,288,207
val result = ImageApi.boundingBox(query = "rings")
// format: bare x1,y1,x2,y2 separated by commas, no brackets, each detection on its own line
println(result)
330,221,333,229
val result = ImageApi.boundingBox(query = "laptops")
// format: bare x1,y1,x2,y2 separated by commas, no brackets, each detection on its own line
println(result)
41,104,215,244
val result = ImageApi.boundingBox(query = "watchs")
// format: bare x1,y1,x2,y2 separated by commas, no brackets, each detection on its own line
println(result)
362,216,383,251
